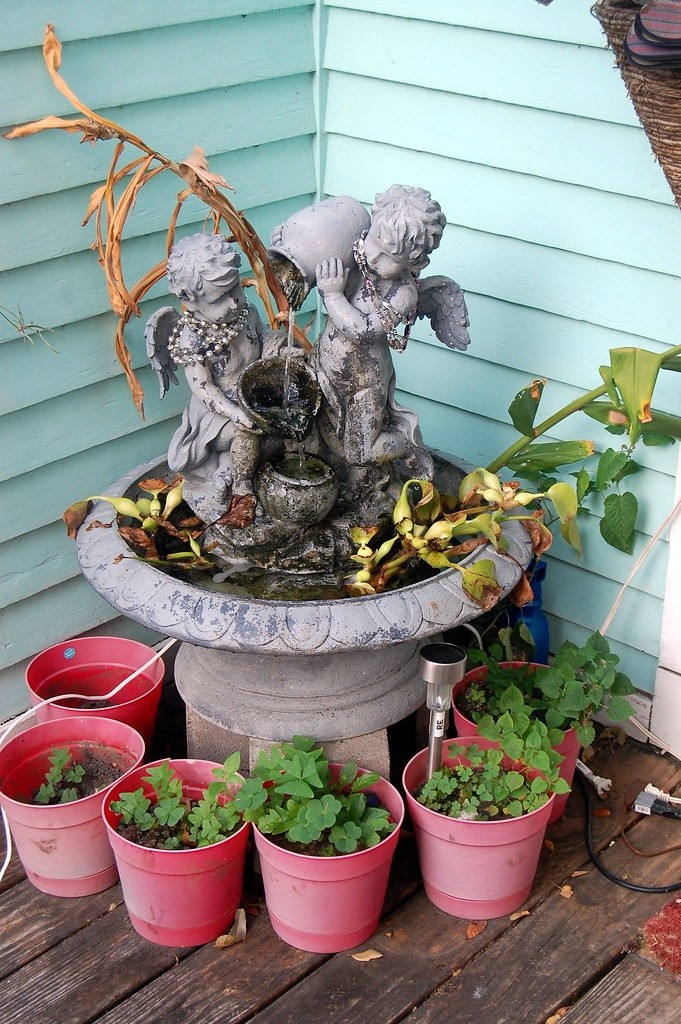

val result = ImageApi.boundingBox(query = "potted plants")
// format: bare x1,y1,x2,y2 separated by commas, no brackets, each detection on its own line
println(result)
451,628,637,825
401,710,572,920
0,716,146,897
233,734,405,953
101,751,252,947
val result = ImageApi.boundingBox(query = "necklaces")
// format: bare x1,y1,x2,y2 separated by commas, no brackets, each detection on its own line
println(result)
352,229,420,354
166,301,249,366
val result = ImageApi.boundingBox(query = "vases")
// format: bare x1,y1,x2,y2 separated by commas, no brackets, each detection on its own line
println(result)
25,634,165,763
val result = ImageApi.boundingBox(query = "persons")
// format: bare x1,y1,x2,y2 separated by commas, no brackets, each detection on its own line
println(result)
269,184,446,484
164,232,309,515
